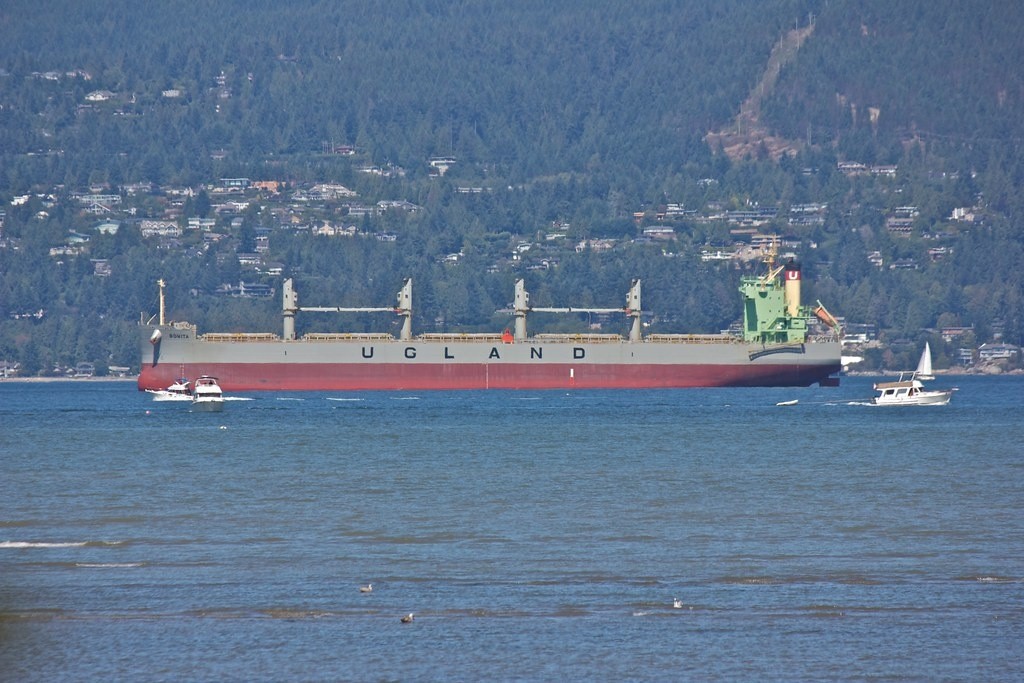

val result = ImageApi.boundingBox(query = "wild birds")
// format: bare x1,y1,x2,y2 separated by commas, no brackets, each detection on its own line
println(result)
401,613,415,623
360,584,373,593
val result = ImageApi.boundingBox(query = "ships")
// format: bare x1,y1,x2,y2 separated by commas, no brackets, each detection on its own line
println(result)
133,226,844,394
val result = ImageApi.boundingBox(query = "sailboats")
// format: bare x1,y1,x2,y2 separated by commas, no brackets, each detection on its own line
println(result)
912,341,937,380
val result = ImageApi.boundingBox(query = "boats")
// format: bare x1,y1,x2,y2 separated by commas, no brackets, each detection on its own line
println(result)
152,377,196,401
187,373,224,412
871,371,953,406
